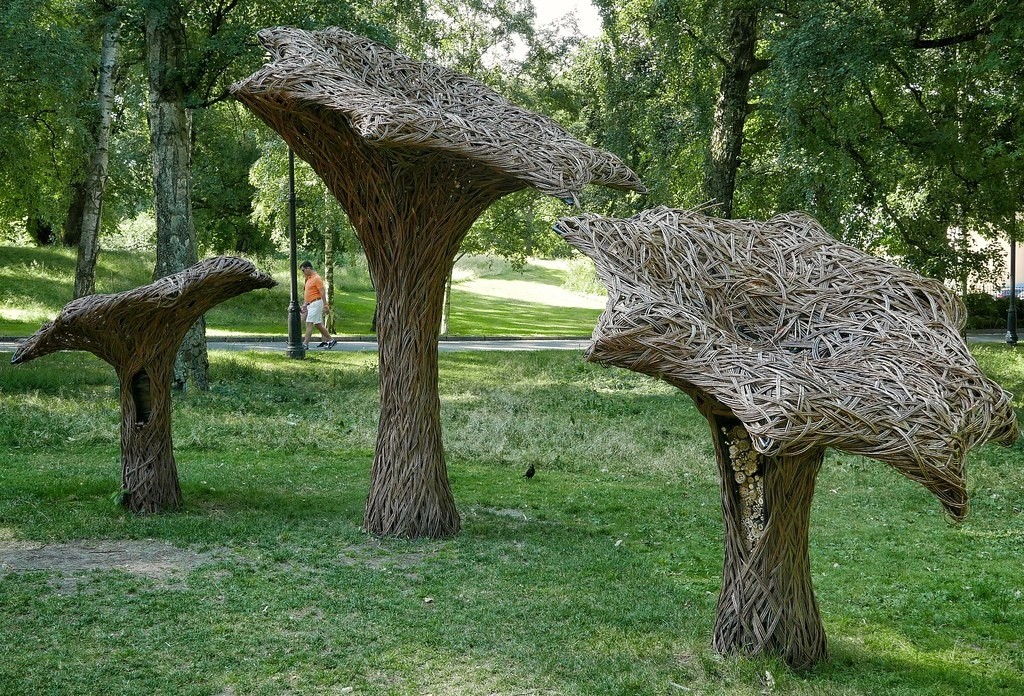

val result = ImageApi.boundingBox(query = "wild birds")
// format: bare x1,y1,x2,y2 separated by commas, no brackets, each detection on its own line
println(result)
525,464,535,479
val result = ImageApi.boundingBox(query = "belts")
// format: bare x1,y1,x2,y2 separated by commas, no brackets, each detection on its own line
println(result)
306,298,321,305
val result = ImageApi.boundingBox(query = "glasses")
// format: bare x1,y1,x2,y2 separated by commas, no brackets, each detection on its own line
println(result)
302,268,306,272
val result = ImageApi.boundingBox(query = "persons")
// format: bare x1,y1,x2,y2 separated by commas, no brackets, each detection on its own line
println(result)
300,261,338,351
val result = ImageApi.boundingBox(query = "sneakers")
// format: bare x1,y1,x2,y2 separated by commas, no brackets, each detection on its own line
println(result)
328,339,337,350
316,341,328,348
302,342,309,350
